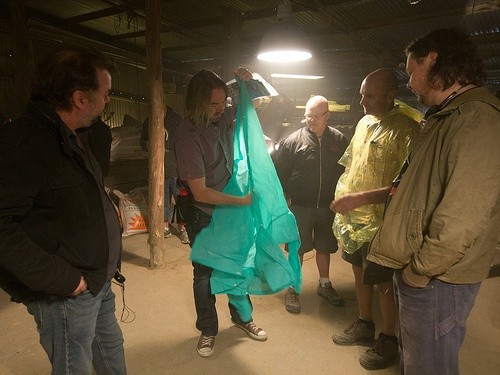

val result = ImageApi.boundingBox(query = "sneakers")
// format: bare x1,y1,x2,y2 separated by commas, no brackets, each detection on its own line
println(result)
197,330,216,356
179,230,191,243
317,279,344,305
360,332,398,368
232,317,267,340
286,287,301,313
332,320,375,344
164,229,171,238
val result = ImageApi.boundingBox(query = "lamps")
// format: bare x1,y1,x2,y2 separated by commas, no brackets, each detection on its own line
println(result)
257,0,312,63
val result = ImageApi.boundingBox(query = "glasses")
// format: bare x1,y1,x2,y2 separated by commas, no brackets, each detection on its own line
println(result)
303,111,328,121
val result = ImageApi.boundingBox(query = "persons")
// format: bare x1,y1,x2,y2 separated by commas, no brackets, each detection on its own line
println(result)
176,68,268,357
366,27,500,375
271,94,348,314
141,96,191,244
0,46,127,375
329,67,422,368
